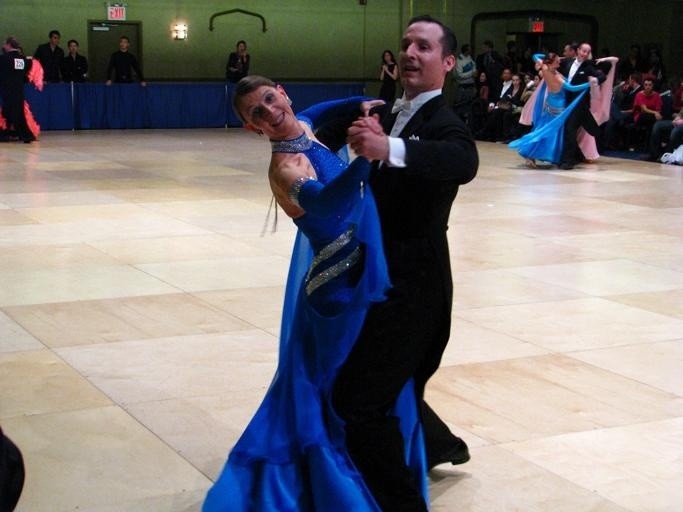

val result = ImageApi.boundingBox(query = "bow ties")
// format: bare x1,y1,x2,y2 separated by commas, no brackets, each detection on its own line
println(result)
392,99,412,115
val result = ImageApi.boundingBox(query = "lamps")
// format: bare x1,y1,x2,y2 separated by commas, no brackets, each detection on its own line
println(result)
174,23,187,41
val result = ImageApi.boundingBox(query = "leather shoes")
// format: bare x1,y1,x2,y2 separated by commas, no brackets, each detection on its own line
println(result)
429,439,468,471
559,161,573,169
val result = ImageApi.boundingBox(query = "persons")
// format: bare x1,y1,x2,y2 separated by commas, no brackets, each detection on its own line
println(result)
32,31,64,84
329,12,480,510
63,40,89,82
0,43,44,140
200,75,386,510
453,36,683,172
376,48,398,101
104,36,148,88
0,36,32,143
225,41,249,80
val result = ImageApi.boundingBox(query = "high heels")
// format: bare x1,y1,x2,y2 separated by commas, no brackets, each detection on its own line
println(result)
524,159,538,169
